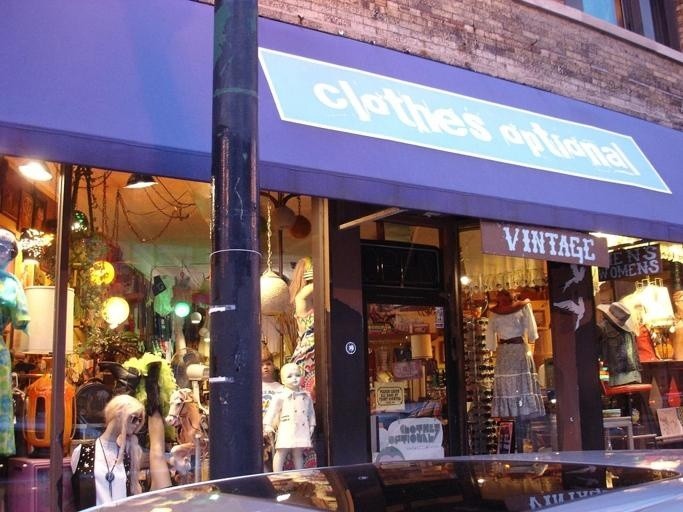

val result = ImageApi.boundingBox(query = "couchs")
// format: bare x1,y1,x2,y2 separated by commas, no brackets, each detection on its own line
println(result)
172,282,192,333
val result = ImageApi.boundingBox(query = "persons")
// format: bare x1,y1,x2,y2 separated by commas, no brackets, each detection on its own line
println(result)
261,257,317,473
71,395,146,511
140,361,172,491
626,304,662,361
597,302,641,386
486,289,547,420
0,229,29,512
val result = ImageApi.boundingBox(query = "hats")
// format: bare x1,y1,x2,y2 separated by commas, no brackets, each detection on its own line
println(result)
73,447,683,512
369,399,448,453
0,368,112,449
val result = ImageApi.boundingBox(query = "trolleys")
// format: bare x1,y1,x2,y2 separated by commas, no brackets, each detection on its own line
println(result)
171,347,208,414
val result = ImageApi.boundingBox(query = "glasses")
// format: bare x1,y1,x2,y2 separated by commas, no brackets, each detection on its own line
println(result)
18,228,45,267
123,173,158,195
17,158,53,186
411,334,433,400
100,295,129,326
89,260,115,288
190,292,202,324
17,286,74,459
174,257,190,318
641,286,675,359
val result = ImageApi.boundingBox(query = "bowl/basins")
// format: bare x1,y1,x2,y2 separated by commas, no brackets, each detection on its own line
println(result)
98,438,119,498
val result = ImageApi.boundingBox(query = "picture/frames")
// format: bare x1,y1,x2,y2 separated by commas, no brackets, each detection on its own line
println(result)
603,416,633,450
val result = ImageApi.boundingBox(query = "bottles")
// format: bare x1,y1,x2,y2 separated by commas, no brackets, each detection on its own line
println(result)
490,289,531,314
0,228,18,259
596,301,633,333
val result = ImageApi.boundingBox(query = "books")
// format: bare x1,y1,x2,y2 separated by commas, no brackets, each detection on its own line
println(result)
8,457,75,512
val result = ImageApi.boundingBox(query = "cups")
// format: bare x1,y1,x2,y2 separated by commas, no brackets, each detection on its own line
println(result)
0,244,17,259
